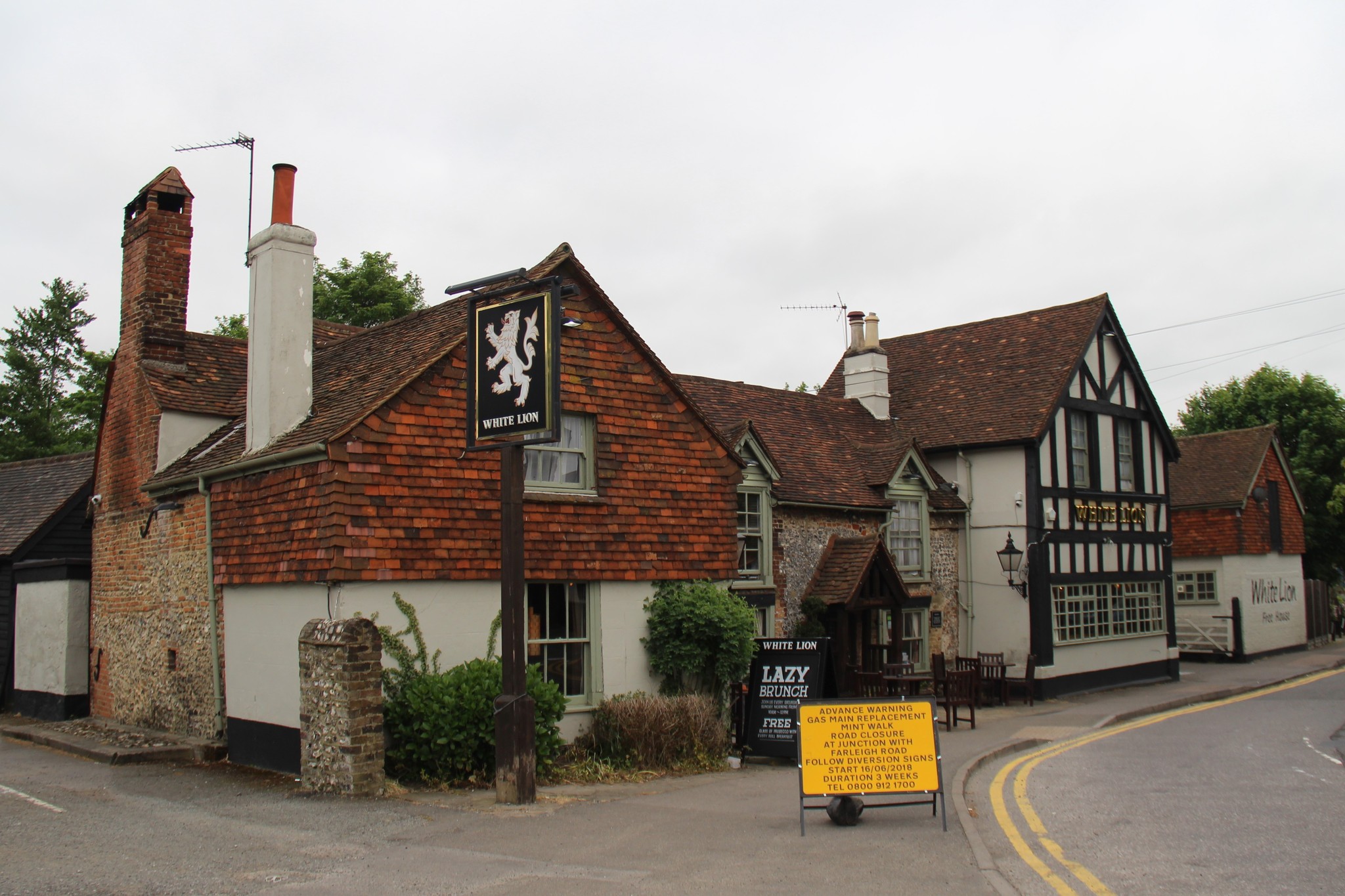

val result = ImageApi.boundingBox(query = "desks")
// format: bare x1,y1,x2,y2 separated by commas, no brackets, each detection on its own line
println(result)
884,675,933,698
967,663,1015,707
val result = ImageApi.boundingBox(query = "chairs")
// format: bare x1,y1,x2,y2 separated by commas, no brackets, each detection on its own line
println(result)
854,651,1036,733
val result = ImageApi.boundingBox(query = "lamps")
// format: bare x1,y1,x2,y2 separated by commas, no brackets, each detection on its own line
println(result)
997,532,1029,600
